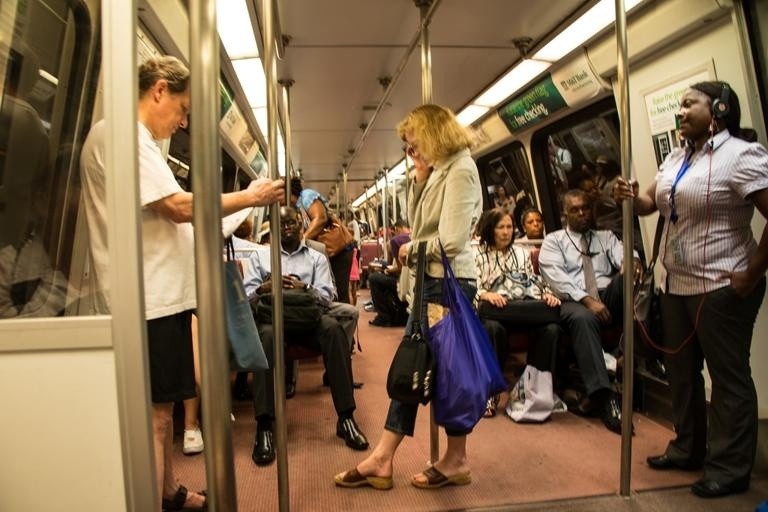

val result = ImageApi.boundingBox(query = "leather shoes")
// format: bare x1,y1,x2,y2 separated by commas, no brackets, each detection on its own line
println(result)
336,416,371,450
234,372,252,401
323,374,362,389
286,376,295,399
692,476,751,496
602,394,636,436
647,455,693,470
252,424,274,467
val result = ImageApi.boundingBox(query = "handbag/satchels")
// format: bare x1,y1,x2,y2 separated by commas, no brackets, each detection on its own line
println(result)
372,306,406,327
634,212,664,323
477,271,561,326
301,197,354,259
256,274,324,329
429,239,510,427
506,366,568,424
387,242,435,406
223,234,270,374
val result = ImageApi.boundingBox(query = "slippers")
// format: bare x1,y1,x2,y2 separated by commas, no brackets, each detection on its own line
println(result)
333,464,396,492
412,466,474,488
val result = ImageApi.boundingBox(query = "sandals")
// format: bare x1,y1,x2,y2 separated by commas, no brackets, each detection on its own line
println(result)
483,393,497,418
161,484,211,512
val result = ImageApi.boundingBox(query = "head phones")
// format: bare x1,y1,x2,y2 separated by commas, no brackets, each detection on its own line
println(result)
711,83,732,120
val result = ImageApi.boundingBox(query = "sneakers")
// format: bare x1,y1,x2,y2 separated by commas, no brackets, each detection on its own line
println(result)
182,428,205,456
364,300,374,305
365,305,376,312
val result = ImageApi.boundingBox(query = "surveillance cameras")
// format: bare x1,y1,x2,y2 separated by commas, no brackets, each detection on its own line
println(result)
512,37,534,62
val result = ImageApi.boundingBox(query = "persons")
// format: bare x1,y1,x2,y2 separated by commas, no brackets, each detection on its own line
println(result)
236,220,254,242
348,241,360,306
518,207,545,250
332,104,484,491
492,184,516,216
369,221,411,327
290,176,355,304
539,189,642,435
242,204,370,467
183,313,236,454
471,208,566,419
344,209,361,263
594,154,622,208
258,221,271,246
81,57,285,512
612,82,767,497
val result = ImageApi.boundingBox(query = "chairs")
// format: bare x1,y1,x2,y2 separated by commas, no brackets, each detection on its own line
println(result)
530,248,541,276
360,242,383,272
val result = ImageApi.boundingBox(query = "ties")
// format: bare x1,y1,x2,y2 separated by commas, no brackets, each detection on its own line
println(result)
581,234,600,304
